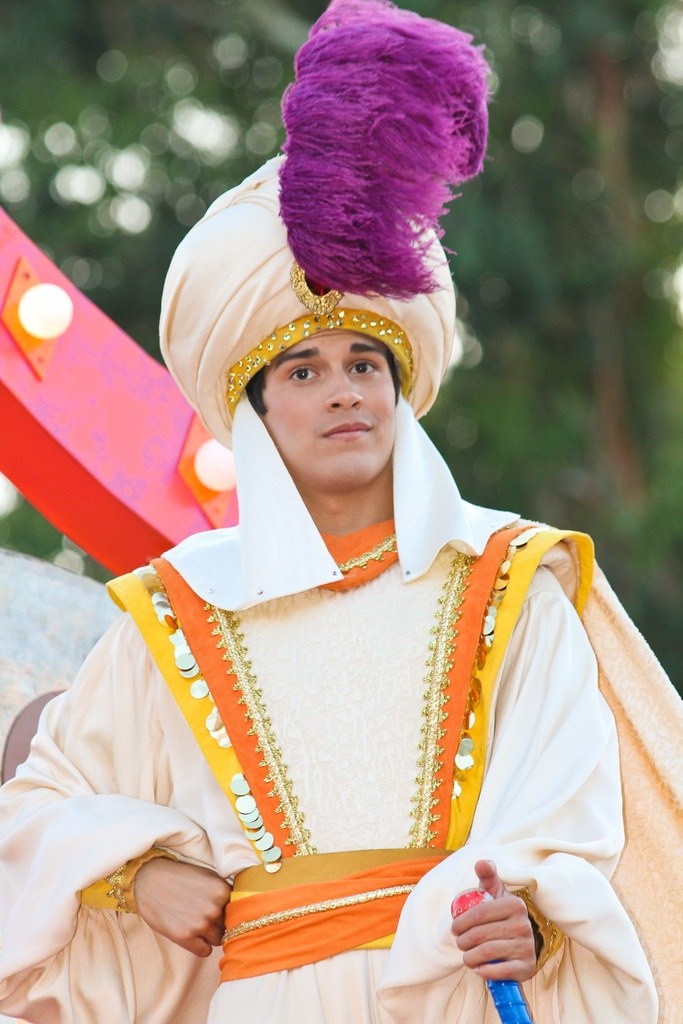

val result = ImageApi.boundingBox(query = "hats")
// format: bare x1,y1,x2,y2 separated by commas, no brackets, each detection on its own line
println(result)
159,0,488,451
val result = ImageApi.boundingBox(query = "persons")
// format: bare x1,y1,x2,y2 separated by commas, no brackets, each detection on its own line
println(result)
1,149,683,1024
